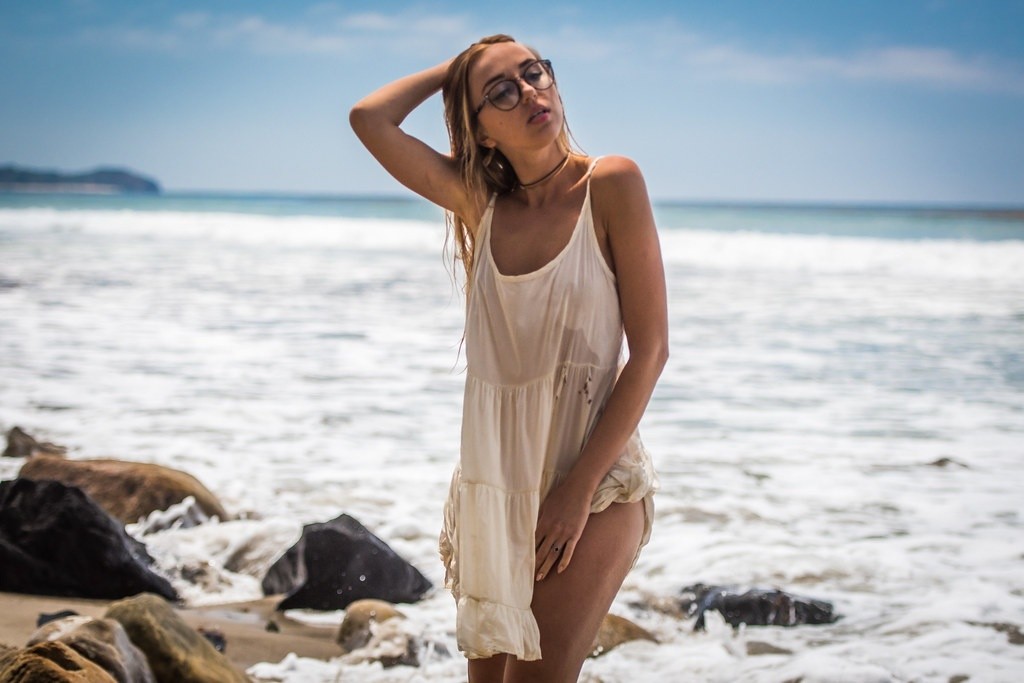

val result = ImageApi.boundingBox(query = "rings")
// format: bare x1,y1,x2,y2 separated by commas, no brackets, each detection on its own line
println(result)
551,545,563,553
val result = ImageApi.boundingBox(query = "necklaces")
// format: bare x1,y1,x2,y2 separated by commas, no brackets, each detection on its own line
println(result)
515,152,570,189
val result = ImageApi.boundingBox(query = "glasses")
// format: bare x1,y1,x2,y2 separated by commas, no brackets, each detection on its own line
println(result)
473,59,555,119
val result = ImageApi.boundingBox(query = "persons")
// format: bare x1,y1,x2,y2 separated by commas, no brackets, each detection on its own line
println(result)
350,35,671,683
